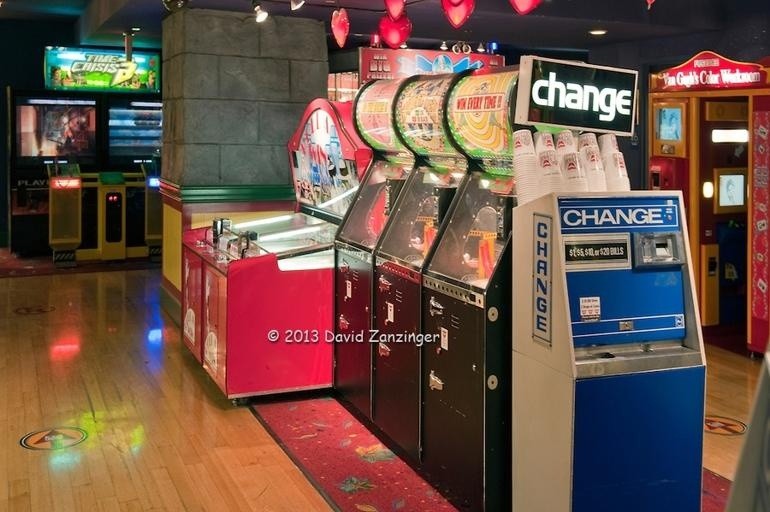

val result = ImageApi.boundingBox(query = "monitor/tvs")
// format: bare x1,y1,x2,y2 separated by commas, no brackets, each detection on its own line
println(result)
100,96,165,166
652,101,687,158
713,165,747,214
10,96,105,168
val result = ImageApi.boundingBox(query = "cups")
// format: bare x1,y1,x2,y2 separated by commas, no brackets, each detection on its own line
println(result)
512,129,632,209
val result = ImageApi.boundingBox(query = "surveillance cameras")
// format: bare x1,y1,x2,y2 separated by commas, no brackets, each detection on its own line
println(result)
159,1,187,13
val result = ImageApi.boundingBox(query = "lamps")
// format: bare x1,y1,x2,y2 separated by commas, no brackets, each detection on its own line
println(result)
252,0,305,23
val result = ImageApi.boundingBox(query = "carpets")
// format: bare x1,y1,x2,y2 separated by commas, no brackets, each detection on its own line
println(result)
247,391,734,512
